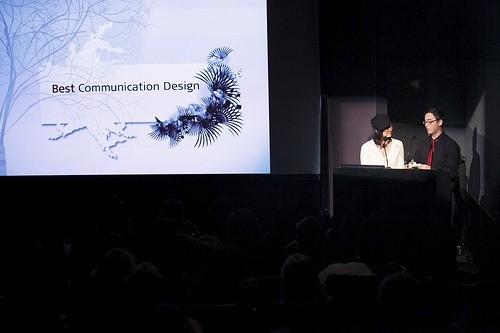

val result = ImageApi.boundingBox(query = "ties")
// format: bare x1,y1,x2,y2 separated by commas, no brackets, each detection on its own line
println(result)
427,141,435,166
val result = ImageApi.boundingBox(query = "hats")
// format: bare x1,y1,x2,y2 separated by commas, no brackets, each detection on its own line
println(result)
371,113,391,132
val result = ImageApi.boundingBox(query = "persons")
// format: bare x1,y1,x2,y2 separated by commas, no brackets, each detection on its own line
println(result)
0,194,500,333
361,113,405,170
404,106,459,178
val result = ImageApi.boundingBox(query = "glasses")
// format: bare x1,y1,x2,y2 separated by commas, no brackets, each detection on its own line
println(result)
423,120,437,125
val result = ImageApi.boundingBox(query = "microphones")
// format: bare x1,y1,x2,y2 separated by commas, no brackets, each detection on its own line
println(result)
407,135,416,169
382,144,392,168
